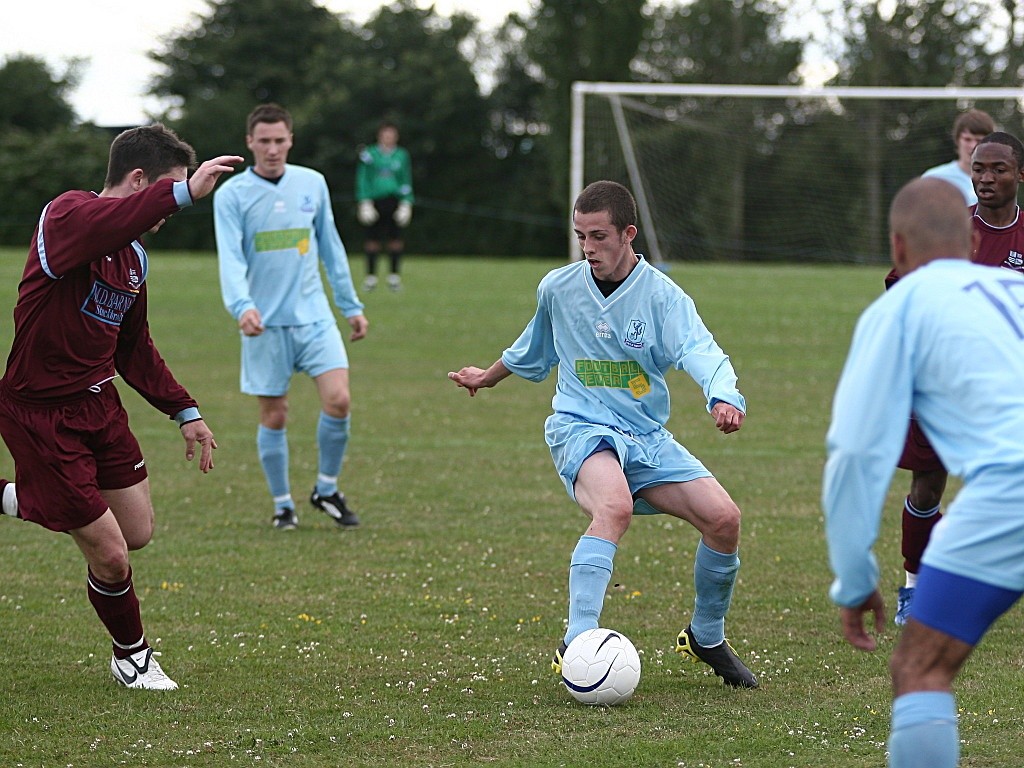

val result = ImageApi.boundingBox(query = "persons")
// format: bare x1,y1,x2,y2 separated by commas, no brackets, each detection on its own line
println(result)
354,120,416,292
213,103,368,530
820,176,1024,768
883,131,1024,625
447,180,759,688
920,108,994,207
0,121,245,690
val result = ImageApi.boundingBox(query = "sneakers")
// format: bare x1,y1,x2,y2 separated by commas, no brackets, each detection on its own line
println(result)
110,647,179,691
550,639,569,674
271,505,299,531
895,584,915,625
675,623,759,689
311,480,360,526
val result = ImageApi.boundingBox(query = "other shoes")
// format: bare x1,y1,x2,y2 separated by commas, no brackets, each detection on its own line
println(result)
362,275,376,291
388,276,400,292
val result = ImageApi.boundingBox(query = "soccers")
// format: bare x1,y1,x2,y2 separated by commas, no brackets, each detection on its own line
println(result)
561,628,643,706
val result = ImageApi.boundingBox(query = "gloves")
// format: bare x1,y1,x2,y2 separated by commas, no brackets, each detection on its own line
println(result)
360,204,380,224
395,204,411,226
